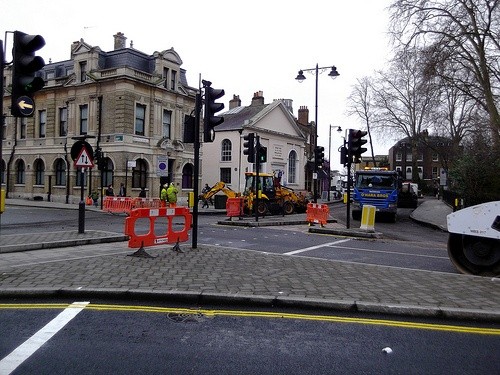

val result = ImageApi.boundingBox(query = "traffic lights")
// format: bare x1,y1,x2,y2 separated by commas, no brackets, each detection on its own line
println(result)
316,146,324,169
351,129,367,164
92,152,108,172
258,147,268,162
9,30,46,117
204,87,223,142
243,133,255,162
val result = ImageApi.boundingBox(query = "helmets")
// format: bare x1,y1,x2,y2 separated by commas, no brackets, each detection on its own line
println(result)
171,182,175,186
164,183,168,188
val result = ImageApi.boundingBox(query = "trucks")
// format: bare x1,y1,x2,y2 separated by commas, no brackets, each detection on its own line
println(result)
352,169,402,223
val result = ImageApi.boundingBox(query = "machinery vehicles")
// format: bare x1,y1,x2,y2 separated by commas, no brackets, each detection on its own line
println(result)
444,200,500,279
198,172,309,215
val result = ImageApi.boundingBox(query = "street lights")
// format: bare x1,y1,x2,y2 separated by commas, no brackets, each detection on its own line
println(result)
327,123,342,203
295,64,340,203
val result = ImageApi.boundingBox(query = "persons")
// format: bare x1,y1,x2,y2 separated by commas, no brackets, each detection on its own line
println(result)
430,179,439,197
258,180,262,190
119,183,126,197
106,185,114,196
138,185,146,197
167,182,178,204
161,183,168,201
201,184,214,209
89,188,99,206
